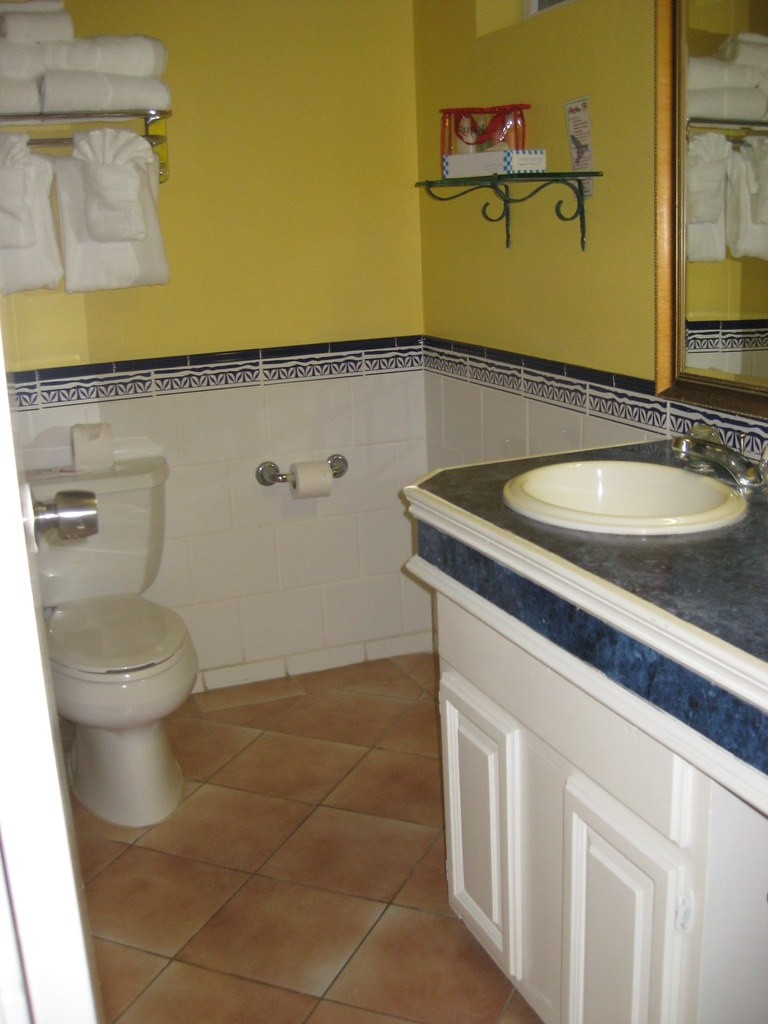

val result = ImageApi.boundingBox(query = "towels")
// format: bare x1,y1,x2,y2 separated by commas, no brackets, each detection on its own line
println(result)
685,31,768,129
52,126,173,293
686,130,767,264
0,1,170,124
0,133,60,294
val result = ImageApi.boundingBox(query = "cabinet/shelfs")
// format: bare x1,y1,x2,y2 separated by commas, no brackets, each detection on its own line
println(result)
439,591,767,1023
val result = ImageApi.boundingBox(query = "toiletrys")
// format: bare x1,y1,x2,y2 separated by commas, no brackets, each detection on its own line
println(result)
458,114,510,154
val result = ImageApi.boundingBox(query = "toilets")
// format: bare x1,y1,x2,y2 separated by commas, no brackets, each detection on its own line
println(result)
24,455,204,829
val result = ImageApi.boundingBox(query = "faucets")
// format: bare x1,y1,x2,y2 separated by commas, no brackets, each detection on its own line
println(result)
673,423,768,492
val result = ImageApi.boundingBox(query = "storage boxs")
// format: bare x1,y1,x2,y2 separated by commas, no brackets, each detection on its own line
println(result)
443,150,547,178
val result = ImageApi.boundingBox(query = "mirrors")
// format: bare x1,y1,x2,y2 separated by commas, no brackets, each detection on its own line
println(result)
674,1,768,399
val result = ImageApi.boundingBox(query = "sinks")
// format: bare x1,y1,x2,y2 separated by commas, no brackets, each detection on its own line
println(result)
501,459,752,537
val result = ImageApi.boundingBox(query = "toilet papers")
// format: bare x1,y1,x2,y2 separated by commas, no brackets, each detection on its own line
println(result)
289,460,335,499
